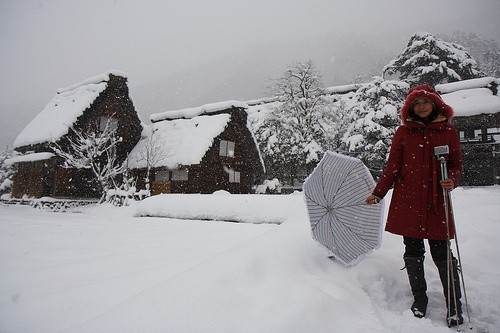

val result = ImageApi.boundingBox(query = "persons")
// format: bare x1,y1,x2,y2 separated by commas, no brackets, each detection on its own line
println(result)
366,85,463,328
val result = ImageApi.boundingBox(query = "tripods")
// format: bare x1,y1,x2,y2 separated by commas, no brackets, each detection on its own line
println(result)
438,156,473,332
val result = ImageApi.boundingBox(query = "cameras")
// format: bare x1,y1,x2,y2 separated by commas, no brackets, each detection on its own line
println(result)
434,146,449,156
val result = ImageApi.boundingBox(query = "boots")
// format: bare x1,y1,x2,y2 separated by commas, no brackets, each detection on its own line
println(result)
435,258,464,326
403,256,429,319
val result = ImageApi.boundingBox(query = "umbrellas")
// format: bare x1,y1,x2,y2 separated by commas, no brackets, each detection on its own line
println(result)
303,150,385,266
